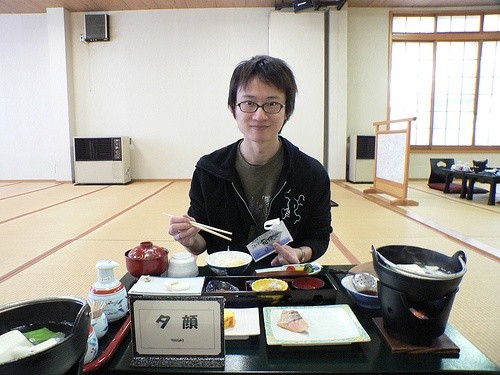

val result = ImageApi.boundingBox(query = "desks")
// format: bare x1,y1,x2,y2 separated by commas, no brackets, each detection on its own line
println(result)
67,264,499,374
443,167,500,205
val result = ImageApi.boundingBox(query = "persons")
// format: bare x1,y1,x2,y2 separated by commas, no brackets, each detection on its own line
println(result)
167,55,333,266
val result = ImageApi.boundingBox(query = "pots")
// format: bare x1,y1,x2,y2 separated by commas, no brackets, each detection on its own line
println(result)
0,296,92,375
472,160,487,173
372,245,467,298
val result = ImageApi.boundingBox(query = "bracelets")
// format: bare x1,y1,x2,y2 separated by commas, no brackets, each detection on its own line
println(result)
300,248,305,263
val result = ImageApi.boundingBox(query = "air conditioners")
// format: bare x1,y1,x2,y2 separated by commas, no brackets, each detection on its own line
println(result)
349,135,376,183
73,135,131,184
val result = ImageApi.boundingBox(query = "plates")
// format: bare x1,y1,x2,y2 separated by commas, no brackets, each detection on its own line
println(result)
223,307,261,340
128,275,206,296
263,304,371,347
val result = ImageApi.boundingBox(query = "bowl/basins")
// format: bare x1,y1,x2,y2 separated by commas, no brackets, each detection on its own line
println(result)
251,278,288,300
291,277,325,290
205,250,253,276
124,241,170,278
167,252,200,278
341,275,382,309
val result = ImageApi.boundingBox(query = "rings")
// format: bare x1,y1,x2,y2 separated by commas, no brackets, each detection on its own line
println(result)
179,234,181,239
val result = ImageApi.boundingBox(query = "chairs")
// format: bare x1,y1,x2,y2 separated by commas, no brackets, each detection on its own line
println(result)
429,158,488,195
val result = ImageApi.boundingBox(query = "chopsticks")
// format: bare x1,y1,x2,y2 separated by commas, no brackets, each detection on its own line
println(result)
162,212,233,242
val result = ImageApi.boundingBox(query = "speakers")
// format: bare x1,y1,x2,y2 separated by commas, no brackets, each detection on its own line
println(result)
84,13,108,40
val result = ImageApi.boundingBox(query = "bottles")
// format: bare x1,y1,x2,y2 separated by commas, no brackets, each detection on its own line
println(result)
82,328,99,365
88,259,128,321
451,161,471,172
90,312,111,339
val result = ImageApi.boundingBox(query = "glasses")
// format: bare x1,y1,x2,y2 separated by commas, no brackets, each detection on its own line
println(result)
234,101,287,114
